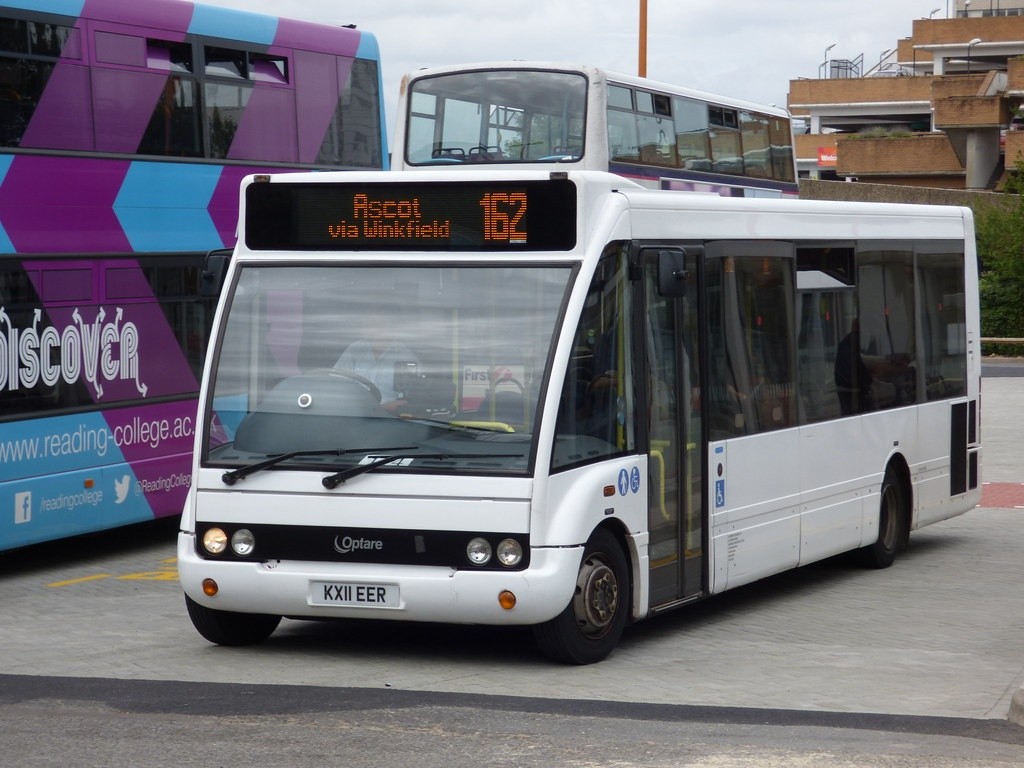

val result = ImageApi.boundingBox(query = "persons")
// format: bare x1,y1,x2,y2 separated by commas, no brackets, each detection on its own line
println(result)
329,302,424,413
835,319,876,417
586,310,683,449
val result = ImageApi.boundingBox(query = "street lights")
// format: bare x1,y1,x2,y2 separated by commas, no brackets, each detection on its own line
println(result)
880,49,891,71
818,61,829,80
964,0,971,17
825,44,836,79
968,38,982,73
929,8,941,19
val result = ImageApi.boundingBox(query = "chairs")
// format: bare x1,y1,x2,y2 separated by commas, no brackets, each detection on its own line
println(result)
745,164,766,179
554,144,583,156
478,377,525,421
432,148,465,161
469,146,502,160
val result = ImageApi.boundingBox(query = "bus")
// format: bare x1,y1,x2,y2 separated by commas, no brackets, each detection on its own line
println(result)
390,60,799,199
177,169,983,668
0,1,396,554
